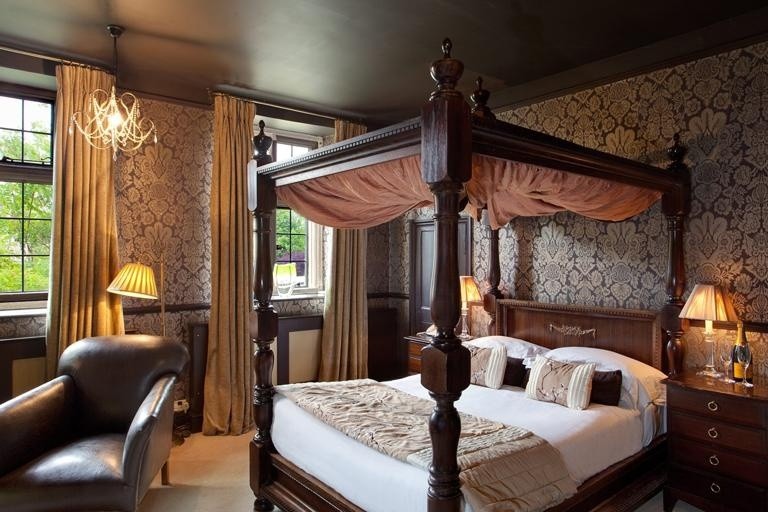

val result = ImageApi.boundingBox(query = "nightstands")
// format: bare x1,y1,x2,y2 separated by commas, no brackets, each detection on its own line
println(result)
658,367,768,512
403,335,434,375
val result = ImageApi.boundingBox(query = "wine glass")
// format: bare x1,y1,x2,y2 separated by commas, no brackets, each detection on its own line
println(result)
720,344,733,383
737,344,754,388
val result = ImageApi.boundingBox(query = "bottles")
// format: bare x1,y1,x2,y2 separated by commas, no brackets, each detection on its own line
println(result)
732,321,752,383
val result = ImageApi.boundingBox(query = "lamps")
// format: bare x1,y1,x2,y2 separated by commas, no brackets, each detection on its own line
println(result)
106,252,166,336
678,283,739,379
459,276,482,341
68,25,157,161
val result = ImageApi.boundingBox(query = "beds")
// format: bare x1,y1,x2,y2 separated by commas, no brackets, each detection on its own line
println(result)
246,40,692,512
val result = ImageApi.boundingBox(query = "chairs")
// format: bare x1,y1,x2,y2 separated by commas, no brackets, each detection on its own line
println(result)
0,335,191,512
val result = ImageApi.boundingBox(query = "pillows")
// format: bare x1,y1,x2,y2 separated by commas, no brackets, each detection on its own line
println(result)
461,342,507,389
525,354,597,412
503,356,530,389
469,335,551,360
590,369,623,406
544,345,669,413
